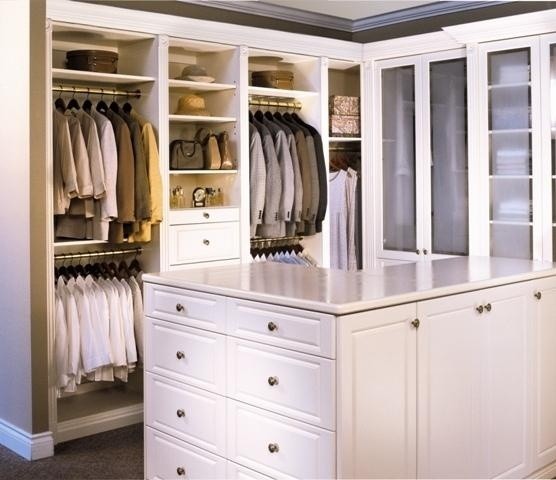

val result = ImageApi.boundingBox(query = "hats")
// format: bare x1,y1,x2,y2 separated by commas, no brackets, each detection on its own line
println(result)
174,96,213,116
174,64,215,83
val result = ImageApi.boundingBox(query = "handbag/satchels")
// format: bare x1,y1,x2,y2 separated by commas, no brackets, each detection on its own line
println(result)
218,130,234,169
171,139,205,170
193,127,221,169
